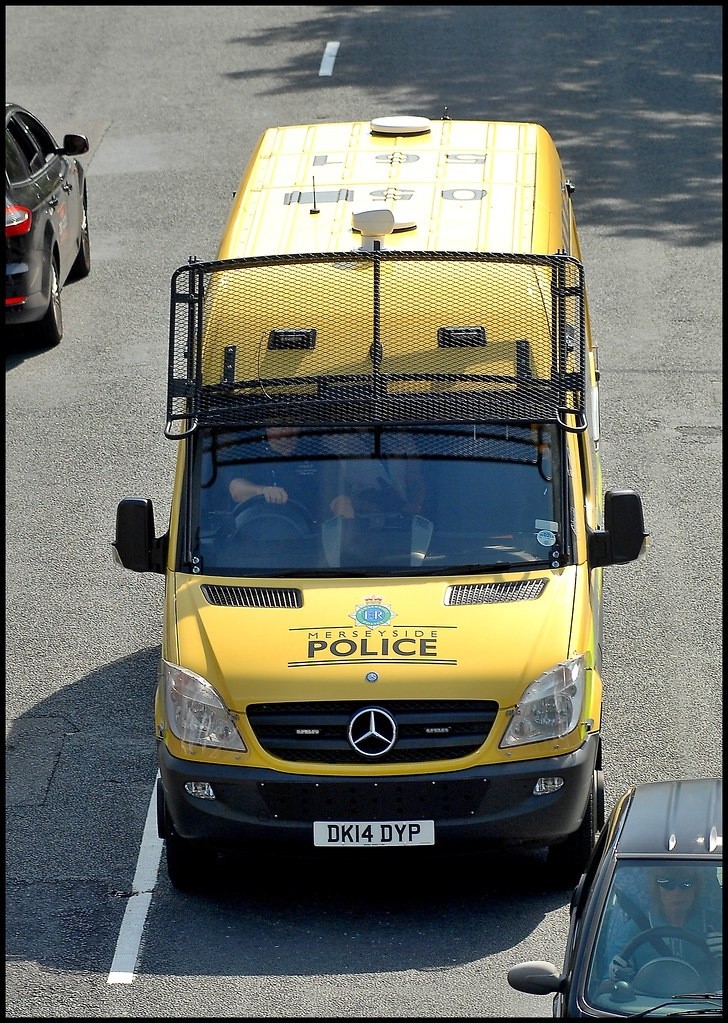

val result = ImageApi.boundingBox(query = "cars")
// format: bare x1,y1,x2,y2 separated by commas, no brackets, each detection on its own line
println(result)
4,101,92,352
505,778,723,1021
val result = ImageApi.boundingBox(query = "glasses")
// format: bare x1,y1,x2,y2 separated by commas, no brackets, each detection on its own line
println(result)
657,875,697,891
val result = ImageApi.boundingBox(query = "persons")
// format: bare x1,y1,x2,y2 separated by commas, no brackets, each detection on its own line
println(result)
604,865,723,991
319,432,428,532
224,427,354,518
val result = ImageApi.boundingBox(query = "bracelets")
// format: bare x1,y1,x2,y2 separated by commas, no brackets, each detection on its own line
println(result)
416,505,425,509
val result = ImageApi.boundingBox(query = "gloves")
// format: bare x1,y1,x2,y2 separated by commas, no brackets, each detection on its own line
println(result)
705,930,722,959
608,953,636,982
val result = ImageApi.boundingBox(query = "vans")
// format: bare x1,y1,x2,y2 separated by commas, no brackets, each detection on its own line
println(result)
112,116,651,900
